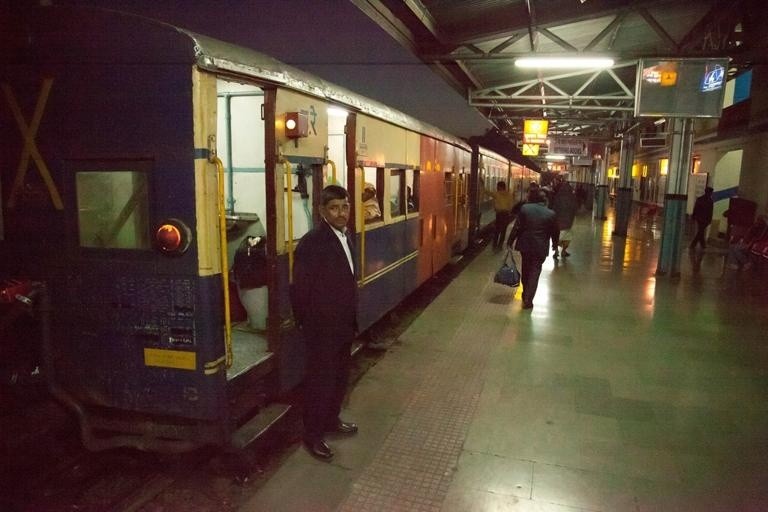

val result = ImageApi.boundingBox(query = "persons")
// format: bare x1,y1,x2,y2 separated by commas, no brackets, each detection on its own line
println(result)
485,174,576,308
362,183,381,220
690,186,713,249
393,186,415,216
292,185,358,461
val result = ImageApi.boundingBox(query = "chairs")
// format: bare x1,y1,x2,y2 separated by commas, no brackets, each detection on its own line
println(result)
727,216,768,265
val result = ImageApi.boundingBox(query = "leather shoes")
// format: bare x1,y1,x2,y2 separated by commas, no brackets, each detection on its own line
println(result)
312,439,333,459
336,423,357,435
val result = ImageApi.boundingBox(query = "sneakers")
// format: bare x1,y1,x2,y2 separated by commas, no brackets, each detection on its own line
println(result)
553,252,570,257
726,262,754,271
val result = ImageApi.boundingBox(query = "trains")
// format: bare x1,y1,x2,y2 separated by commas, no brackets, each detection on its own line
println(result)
0,4,551,468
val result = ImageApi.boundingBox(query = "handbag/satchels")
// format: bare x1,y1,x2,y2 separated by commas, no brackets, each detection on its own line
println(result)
494,250,520,287
